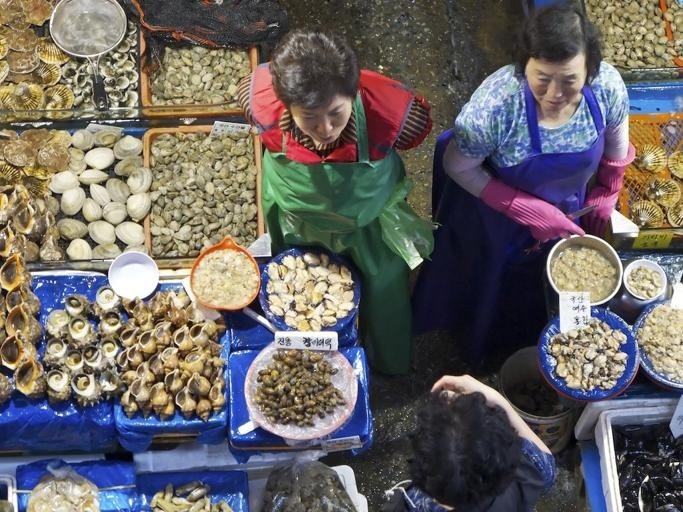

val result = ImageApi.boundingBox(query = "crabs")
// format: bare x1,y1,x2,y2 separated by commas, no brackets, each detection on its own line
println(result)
629,144,682,228
0,1,74,195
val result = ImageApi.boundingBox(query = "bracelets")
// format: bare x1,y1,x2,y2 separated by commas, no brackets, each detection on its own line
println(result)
546,233,623,307
107,251,159,301
189,236,263,312
621,259,668,301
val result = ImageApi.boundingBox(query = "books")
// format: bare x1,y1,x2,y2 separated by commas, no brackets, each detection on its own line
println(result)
593,404,683,512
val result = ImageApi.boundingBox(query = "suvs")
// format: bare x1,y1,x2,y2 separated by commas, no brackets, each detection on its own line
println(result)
498,344,584,456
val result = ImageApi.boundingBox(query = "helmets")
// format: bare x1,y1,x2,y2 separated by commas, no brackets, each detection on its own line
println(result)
535,304,639,403
627,296,683,393
261,243,364,339
241,337,358,441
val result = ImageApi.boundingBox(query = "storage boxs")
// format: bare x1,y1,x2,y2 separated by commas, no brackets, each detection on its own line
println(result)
593,404,683,512
0,0,372,512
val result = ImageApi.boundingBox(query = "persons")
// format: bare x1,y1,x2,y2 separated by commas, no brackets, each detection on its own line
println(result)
380,374,555,512
409,6,636,373
237,25,435,377
